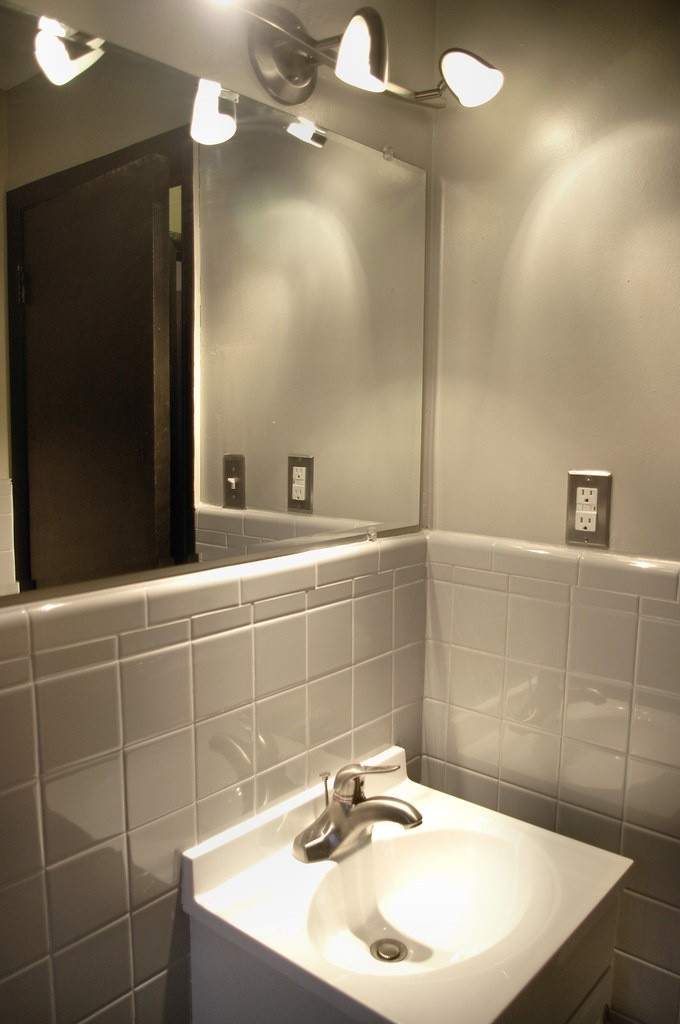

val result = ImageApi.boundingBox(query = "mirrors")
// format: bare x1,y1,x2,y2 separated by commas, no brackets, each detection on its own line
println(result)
0,0,428,598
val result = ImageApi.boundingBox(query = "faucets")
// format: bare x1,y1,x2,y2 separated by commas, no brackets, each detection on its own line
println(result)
294,762,423,864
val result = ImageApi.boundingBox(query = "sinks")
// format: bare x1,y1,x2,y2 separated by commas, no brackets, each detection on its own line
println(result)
297,823,548,978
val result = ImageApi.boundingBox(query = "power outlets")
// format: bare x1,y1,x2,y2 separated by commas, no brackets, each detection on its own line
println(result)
566,468,610,549
285,456,314,514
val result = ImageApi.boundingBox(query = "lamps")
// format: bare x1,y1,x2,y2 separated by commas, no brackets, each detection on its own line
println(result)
280,116,328,148
190,74,241,145
411,47,504,108
251,3,392,106
